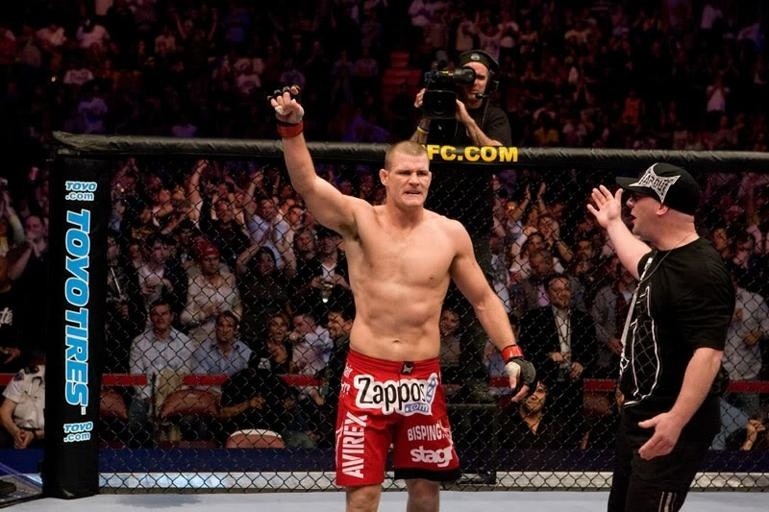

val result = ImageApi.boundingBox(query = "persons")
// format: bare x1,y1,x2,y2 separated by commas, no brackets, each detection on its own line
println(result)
440,308,476,407
218,351,294,445
395,51,509,404
190,311,252,399
696,0,763,287
517,4,694,146
287,231,351,312
2,361,47,451
2,2,47,352
326,308,353,402
268,85,536,510
401,2,517,68
709,379,754,471
498,376,573,470
130,304,192,447
593,259,635,377
493,163,629,304
237,249,290,312
251,314,289,374
53,2,394,143
282,315,331,397
524,275,591,417
586,160,736,511
724,263,763,378
184,244,240,321
715,394,769,472
110,155,268,244
130,236,184,320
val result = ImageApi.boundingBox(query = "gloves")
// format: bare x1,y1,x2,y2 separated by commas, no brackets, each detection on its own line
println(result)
502,345,540,398
266,86,304,137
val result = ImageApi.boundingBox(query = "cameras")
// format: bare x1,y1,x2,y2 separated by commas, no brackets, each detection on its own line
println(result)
423,67,476,120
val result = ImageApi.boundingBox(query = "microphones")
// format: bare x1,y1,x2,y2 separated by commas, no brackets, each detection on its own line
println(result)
456,50,500,99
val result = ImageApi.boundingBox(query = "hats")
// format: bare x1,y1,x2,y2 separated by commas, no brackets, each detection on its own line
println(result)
616,163,701,213
460,52,491,70
